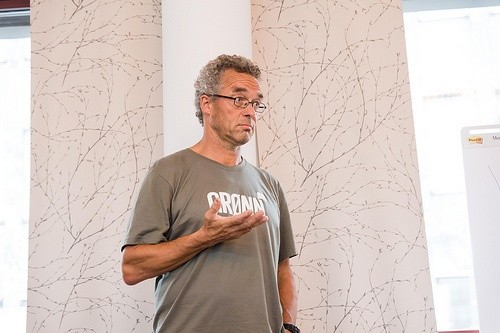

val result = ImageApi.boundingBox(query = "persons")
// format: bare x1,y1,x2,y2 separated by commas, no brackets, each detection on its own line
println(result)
121,54,302,333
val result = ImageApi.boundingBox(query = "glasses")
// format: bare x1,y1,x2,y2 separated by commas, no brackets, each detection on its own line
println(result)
204,93,267,113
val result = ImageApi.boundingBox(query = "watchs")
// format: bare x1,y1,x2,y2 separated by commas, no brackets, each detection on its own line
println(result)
283,323,301,333
